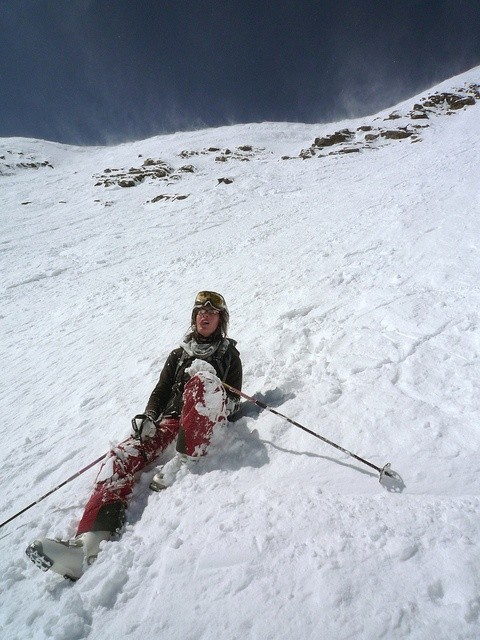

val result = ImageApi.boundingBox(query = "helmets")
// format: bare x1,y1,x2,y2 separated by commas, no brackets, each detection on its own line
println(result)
191,291,229,327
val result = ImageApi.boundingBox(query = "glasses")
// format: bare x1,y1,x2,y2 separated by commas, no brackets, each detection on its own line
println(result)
194,291,229,309
199,309,217,314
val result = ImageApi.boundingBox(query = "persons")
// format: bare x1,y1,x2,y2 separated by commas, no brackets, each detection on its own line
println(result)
28,289,243,581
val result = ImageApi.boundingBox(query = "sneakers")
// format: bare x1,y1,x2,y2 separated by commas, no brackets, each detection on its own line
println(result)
26,530,112,582
149,452,201,492
